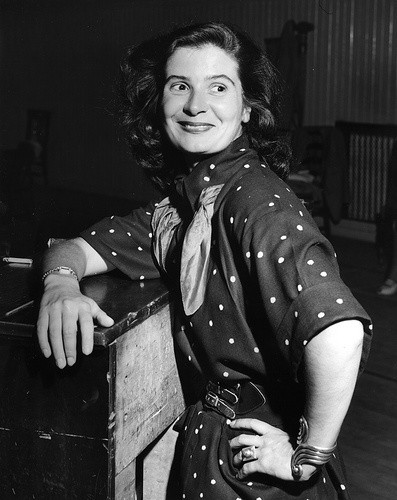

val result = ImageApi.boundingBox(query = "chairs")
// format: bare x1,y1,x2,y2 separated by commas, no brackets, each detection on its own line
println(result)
18,108,49,184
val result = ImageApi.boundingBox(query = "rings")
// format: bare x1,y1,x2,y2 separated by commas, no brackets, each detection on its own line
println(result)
241,446,255,461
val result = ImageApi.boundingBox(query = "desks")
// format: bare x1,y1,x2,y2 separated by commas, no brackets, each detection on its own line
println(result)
0,260,186,500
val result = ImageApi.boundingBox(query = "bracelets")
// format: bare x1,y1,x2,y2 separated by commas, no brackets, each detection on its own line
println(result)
291,415,337,477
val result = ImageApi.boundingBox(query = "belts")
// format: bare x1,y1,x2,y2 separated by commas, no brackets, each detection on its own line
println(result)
204,376,307,421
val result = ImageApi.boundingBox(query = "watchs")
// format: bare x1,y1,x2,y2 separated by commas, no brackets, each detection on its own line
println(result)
41,266,80,282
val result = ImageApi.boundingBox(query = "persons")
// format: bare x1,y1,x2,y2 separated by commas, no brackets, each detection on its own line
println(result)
37,20,374,500
375,136,396,295
